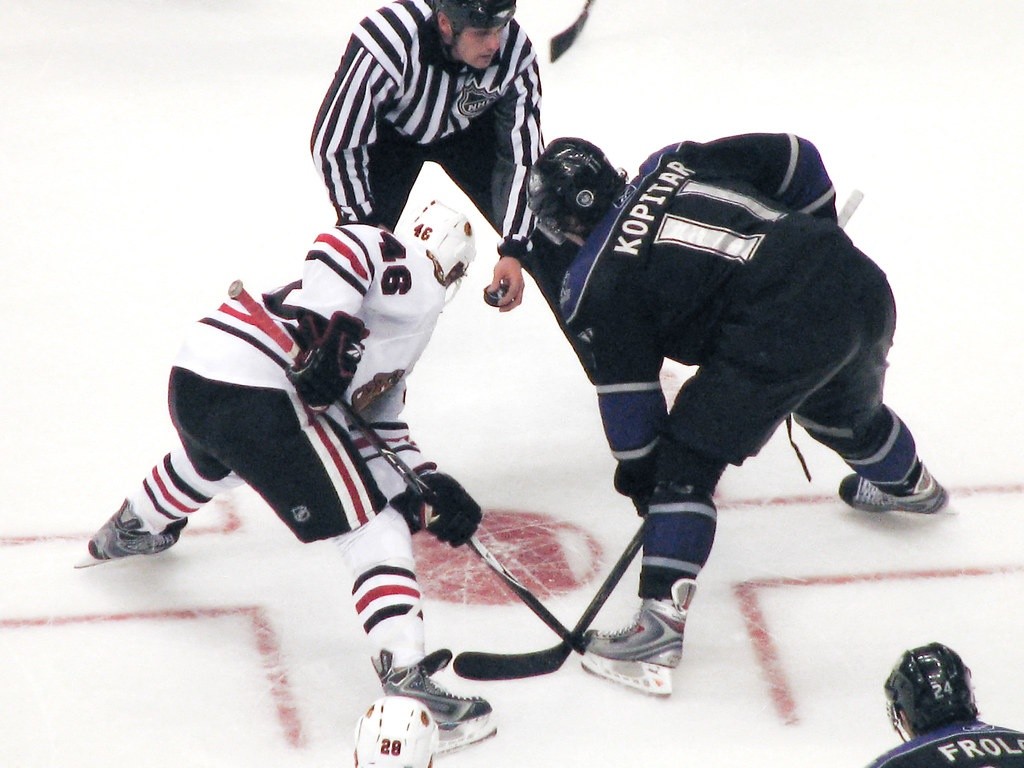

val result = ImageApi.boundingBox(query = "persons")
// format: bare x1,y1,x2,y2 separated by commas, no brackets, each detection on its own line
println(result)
309,0,595,385
524,134,947,696
859,641,1024,768
86,198,499,767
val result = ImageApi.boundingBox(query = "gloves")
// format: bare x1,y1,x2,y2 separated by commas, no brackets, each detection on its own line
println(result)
612,433,660,517
388,467,482,548
285,310,371,408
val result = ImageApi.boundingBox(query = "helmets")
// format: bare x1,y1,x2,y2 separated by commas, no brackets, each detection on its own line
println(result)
525,137,627,239
403,199,477,281
351,696,438,768
884,642,980,733
424,0,517,34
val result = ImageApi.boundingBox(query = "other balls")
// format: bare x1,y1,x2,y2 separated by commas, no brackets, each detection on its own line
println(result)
483,284,511,308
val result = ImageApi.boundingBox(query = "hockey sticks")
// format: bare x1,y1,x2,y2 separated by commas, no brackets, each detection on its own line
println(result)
227,279,599,656
452,189,865,680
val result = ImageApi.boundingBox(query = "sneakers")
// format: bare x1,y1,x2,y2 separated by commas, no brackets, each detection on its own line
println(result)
581,578,697,694
370,649,498,758
73,497,188,569
838,458,959,516
